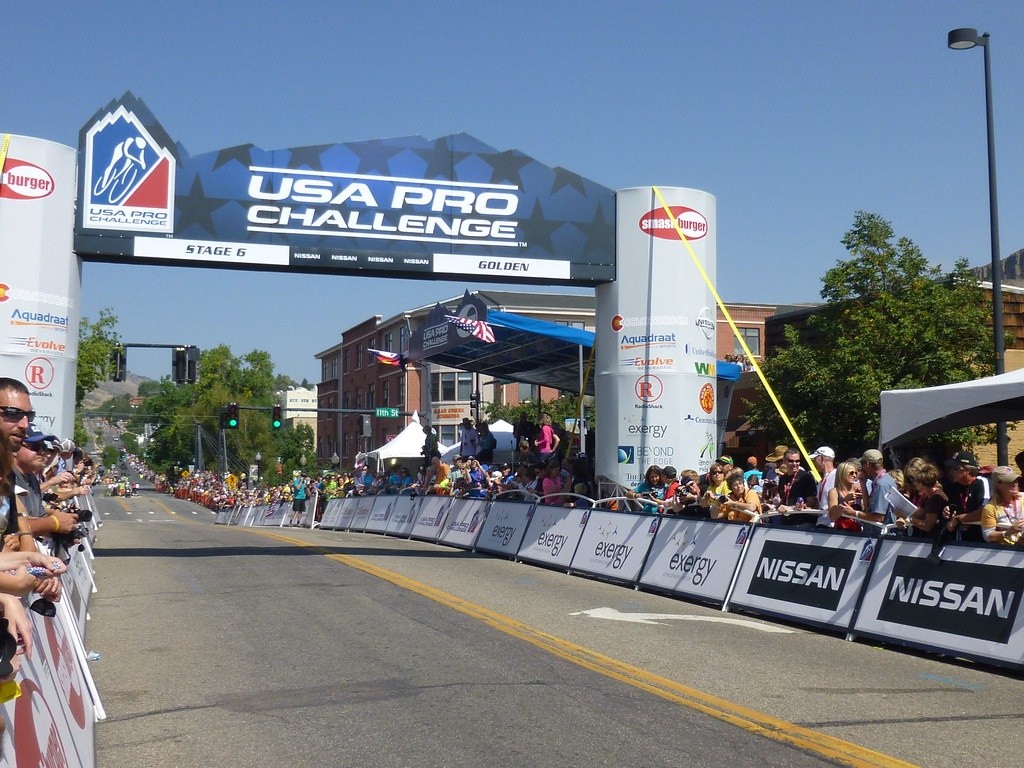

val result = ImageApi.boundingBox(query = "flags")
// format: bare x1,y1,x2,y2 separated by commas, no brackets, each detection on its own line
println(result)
371,350,400,366
448,316,495,343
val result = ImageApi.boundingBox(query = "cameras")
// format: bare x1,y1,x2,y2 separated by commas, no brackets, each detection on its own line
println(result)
80,458,93,466
719,496,728,503
71,524,89,539
67,502,92,522
651,491,658,498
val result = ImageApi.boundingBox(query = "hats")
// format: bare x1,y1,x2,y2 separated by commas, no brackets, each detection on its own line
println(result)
715,455,733,466
44,456,58,473
500,462,511,469
452,454,462,460
858,449,883,464
661,465,676,478
460,418,473,425
44,440,54,450
23,423,54,442
943,451,976,467
681,476,692,485
59,438,76,452
991,465,1022,485
809,446,835,459
765,445,788,462
51,435,63,450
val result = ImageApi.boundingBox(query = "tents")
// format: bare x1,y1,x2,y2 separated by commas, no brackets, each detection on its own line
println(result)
440,418,518,466
878,367,1024,452
355,418,448,473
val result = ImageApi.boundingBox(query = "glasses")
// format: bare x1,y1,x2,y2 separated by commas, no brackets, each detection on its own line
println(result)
848,471,857,475
362,469,367,471
536,470,541,474
21,441,47,452
400,470,406,474
0,406,35,422
687,481,695,489
54,465,59,470
784,457,800,463
711,470,724,475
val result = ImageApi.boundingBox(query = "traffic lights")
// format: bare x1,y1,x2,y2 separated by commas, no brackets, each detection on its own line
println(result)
227,402,239,429
271,404,281,430
470,393,477,416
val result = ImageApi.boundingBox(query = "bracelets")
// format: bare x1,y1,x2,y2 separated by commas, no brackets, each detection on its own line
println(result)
633,493,637,498
20,532,32,536
855,511,859,517
1002,531,1007,537
51,515,59,532
955,516,961,525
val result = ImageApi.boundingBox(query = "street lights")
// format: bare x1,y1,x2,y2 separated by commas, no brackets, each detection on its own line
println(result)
948,28,1008,470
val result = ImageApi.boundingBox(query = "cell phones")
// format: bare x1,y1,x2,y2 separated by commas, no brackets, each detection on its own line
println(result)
948,504,957,515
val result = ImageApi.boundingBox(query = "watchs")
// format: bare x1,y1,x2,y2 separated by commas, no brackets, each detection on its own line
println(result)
17,512,27,517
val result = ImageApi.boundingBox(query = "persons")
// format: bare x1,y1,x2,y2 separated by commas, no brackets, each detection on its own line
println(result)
459,417,494,466
626,446,1024,547
420,425,437,467
94,452,174,497
513,410,562,464
0,378,94,733
173,468,294,510
289,455,595,527
